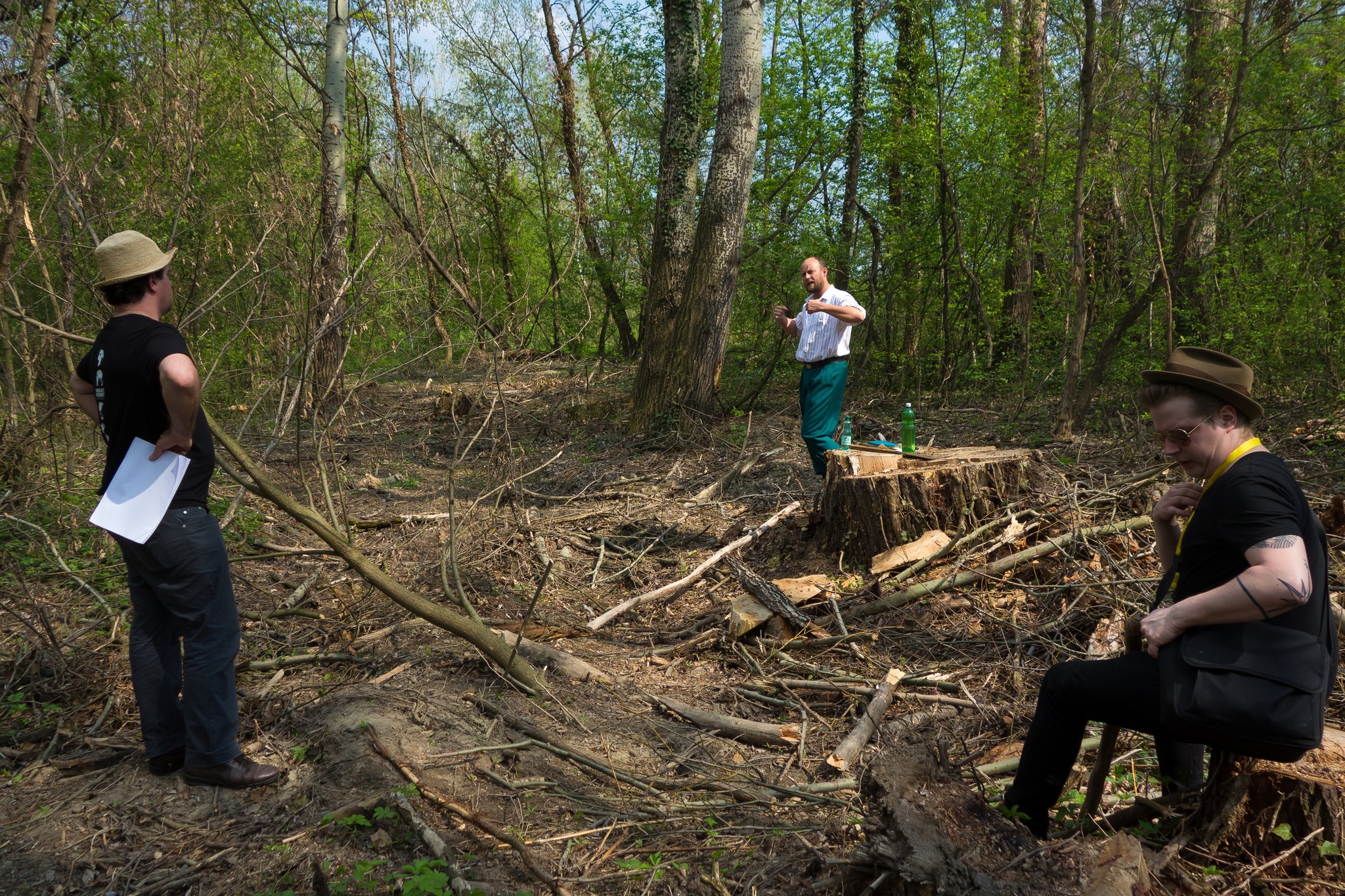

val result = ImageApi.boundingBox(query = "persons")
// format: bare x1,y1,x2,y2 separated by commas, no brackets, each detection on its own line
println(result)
996,347,1335,853
774,257,866,480
69,232,281,792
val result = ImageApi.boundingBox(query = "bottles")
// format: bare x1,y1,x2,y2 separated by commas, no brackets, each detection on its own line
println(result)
841,416,852,451
901,403,915,453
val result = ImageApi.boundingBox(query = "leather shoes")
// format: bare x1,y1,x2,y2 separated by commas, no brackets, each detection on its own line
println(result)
150,745,186,776
183,754,280,789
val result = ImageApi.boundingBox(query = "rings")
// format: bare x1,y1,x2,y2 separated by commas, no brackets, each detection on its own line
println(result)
776,316,778,319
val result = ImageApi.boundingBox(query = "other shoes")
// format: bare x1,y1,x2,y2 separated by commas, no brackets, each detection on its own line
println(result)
994,804,1050,841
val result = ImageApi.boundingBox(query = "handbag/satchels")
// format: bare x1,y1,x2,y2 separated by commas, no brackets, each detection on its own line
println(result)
1159,620,1330,763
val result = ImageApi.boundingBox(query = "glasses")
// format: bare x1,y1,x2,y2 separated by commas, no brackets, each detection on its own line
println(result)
1150,408,1219,450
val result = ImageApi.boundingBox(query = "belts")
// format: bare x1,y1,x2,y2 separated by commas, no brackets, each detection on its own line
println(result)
805,356,845,369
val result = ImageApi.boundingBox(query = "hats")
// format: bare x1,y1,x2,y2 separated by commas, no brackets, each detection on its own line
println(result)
1141,346,1264,421
93,230,178,289
